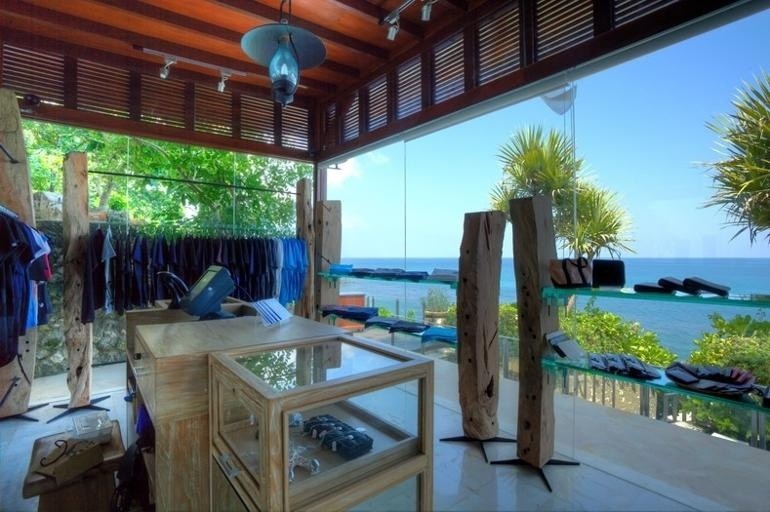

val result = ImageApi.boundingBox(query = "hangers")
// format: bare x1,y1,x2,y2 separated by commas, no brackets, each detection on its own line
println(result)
95,219,298,239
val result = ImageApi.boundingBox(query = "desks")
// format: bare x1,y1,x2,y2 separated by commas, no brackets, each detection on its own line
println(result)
124,295,352,511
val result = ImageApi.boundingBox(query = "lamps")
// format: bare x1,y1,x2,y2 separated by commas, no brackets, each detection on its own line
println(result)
159,55,177,79
240,0,327,108
386,15,400,41
217,70,231,92
422,0,440,21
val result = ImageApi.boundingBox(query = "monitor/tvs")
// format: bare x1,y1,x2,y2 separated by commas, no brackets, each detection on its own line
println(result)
179,265,236,321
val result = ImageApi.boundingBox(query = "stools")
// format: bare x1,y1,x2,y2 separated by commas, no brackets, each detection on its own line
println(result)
23,420,125,512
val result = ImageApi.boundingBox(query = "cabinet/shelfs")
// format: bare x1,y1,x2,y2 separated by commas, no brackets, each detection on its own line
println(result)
208,335,434,512
